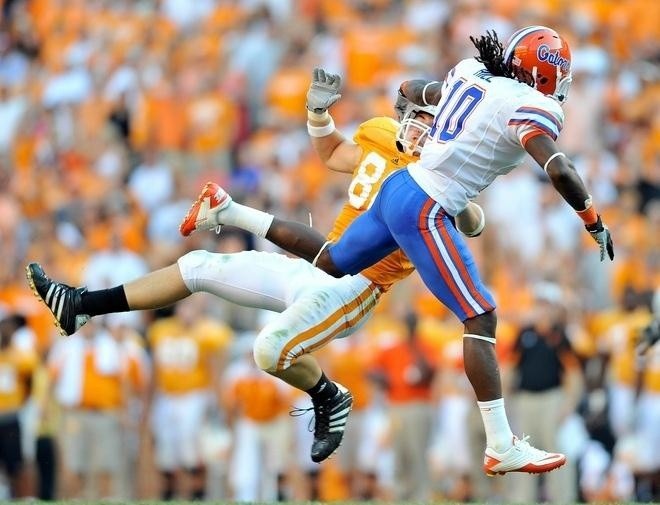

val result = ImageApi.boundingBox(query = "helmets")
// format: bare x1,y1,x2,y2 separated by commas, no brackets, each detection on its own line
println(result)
394,106,438,158
501,26,572,104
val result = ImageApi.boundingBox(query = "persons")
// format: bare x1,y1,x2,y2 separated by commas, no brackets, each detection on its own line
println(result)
2,0,660,505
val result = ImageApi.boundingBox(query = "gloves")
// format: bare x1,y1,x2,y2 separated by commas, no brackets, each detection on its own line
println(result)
585,215,614,262
395,91,409,123
304,68,342,114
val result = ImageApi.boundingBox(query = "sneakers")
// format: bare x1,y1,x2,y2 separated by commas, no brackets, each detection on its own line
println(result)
483,435,566,477
290,380,353,463
25,262,89,336
179,181,231,237
